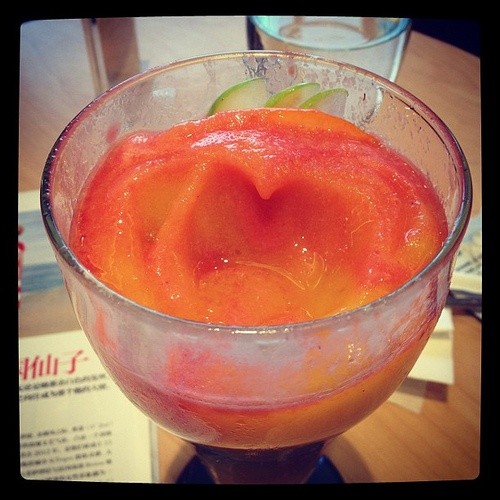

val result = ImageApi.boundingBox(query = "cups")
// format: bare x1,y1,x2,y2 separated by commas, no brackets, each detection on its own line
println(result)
39,49,472,485
248,16,412,130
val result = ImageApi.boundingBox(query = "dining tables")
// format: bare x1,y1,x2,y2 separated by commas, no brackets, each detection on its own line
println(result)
18,16,482,483
